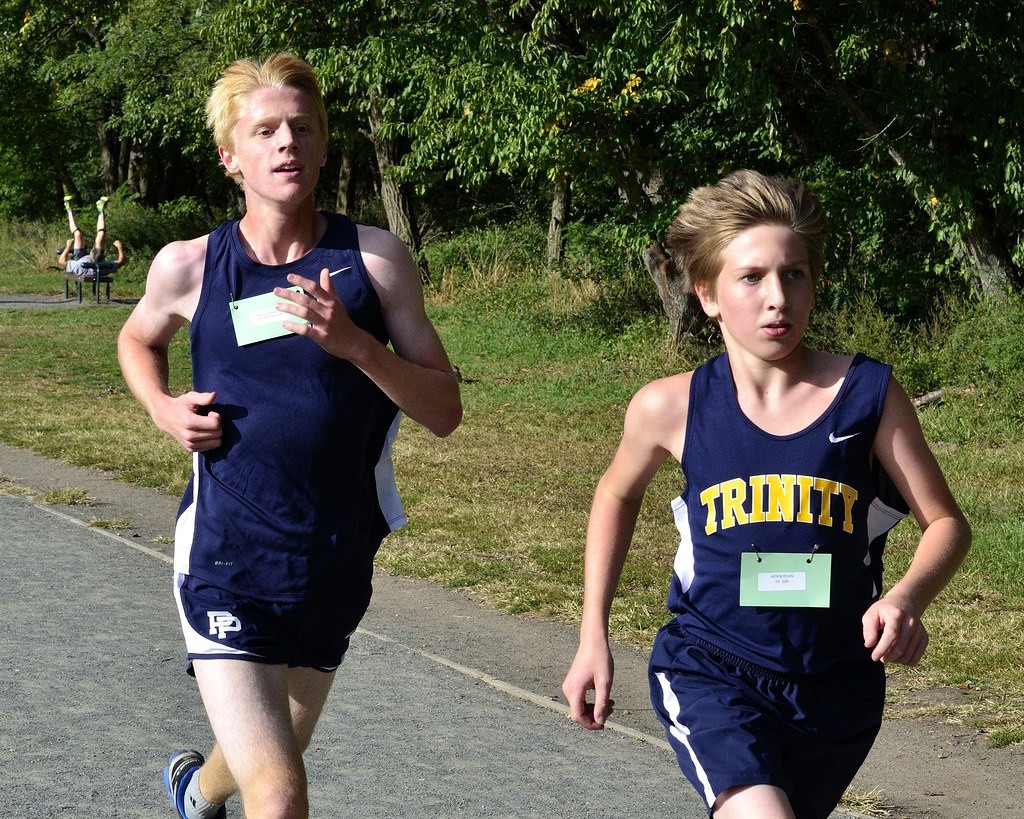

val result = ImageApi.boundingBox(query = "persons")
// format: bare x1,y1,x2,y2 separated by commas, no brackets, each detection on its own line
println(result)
58,195,125,275
562,168,971,819
117,53,462,819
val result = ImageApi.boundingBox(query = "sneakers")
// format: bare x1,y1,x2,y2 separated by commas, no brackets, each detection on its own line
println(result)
64,196,74,210
96,196,110,210
164,748,226,819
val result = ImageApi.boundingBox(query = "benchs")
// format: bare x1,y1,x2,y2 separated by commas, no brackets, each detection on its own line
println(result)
64,272,113,304
